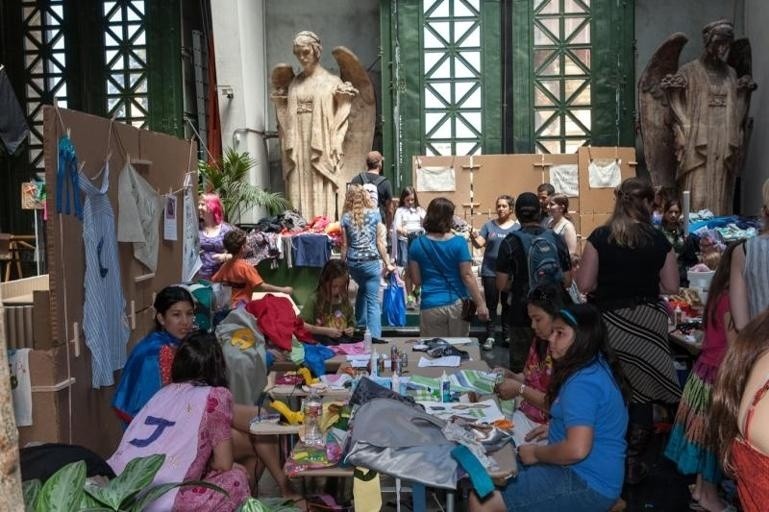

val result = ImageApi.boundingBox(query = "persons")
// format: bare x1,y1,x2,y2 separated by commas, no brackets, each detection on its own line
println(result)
270,30,359,223
658,16,758,218
106,179,767,510
351,150,398,246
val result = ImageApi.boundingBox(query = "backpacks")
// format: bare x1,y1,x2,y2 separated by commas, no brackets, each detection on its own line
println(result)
359,173,387,207
509,228,566,289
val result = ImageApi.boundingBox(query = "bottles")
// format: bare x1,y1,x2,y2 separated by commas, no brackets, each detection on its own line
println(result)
391,370,400,393
440,368,450,402
370,347,377,376
303,387,324,444
673,302,683,328
376,351,385,377
363,326,372,353
399,349,409,374
390,343,397,372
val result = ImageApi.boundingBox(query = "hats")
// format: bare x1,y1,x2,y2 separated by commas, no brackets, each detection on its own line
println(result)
515,192,540,216
367,152,384,163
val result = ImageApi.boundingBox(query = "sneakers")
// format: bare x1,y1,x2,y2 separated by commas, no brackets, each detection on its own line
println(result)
372,337,388,344
353,331,366,336
483,338,495,350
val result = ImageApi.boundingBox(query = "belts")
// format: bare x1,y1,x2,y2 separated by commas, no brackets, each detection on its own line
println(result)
344,256,380,262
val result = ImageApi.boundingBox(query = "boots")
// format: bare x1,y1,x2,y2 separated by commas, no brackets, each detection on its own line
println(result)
625,422,652,485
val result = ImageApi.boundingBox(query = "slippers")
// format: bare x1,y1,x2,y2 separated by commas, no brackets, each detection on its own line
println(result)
689,497,737,512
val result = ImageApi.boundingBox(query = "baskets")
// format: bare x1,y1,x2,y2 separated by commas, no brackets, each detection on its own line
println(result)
349,376,415,406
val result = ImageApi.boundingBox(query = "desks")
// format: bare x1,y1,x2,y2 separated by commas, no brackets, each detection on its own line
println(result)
665,323,704,363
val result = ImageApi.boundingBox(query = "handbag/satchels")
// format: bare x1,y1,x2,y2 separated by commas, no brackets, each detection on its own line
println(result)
343,397,459,491
461,297,477,322
382,272,407,327
